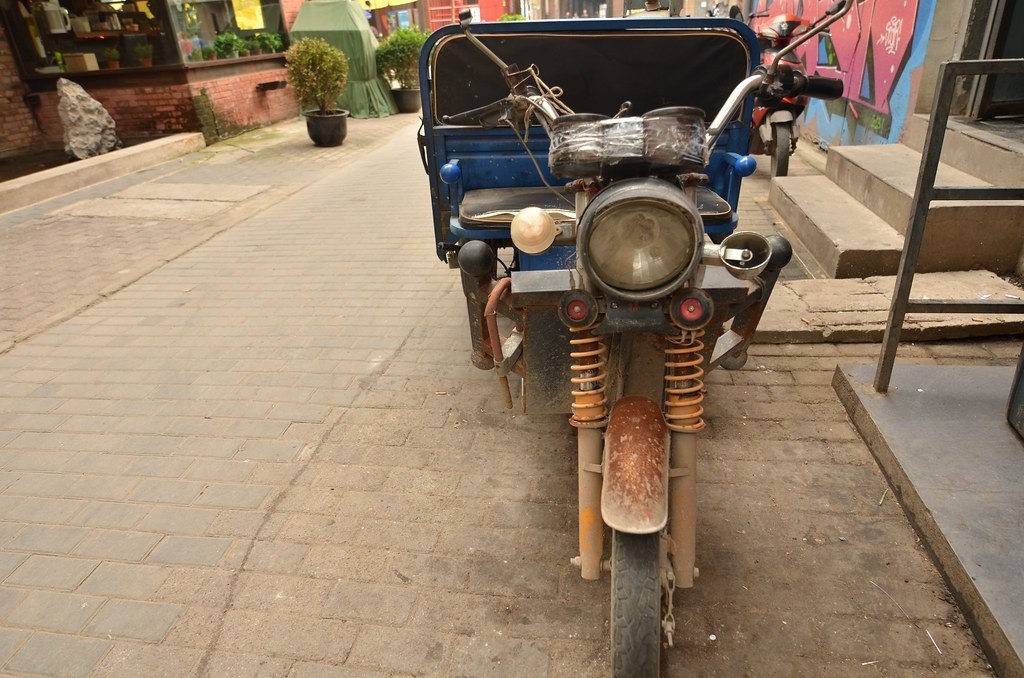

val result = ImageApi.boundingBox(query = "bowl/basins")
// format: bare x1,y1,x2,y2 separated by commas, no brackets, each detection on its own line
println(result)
70,17,90,33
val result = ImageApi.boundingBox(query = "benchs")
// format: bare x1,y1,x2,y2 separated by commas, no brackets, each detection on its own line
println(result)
440,151,756,240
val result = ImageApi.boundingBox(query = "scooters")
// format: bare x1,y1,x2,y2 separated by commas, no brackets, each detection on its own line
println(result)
727,0,848,176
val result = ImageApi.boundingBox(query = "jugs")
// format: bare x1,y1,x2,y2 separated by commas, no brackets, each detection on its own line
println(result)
41,2,71,33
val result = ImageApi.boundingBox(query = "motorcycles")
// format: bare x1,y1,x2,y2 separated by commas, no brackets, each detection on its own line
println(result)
412,0,857,677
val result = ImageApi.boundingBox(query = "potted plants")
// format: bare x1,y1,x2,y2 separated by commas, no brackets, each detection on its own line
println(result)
103,49,120,68
134,43,152,67
376,24,438,113
284,36,351,146
193,32,283,61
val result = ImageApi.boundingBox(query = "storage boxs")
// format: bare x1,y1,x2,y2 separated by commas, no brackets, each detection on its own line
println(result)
64,53,99,73
